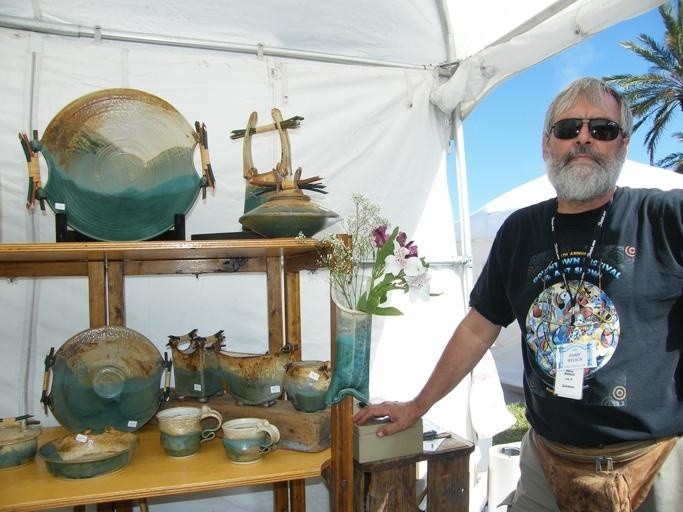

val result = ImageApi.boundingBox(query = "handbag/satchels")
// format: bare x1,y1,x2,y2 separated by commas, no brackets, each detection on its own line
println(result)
528,432,679,511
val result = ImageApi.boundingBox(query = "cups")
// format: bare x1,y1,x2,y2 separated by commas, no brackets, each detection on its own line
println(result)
221,417,280,464
155,406,222,456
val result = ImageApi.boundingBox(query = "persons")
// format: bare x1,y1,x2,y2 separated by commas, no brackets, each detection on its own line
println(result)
348,74,682,511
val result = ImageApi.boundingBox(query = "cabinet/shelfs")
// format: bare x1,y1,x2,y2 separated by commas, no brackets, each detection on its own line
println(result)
0,235,373,512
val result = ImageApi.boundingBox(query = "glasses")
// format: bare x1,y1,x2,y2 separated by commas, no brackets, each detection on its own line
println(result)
547,116,626,142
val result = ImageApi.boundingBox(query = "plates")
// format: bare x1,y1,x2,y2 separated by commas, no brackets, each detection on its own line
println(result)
15,88,215,243
38,325,172,436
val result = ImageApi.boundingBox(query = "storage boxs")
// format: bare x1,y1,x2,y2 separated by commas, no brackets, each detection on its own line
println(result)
352,413,422,464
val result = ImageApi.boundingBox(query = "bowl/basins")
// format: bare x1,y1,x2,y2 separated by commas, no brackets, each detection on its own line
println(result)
37,429,138,479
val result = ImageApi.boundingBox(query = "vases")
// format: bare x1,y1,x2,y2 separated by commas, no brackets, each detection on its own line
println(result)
323,275,376,405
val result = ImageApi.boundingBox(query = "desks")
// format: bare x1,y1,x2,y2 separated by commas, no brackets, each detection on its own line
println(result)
346,431,474,512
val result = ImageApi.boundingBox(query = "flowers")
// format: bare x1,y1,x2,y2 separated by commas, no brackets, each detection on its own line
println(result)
295,192,428,317
